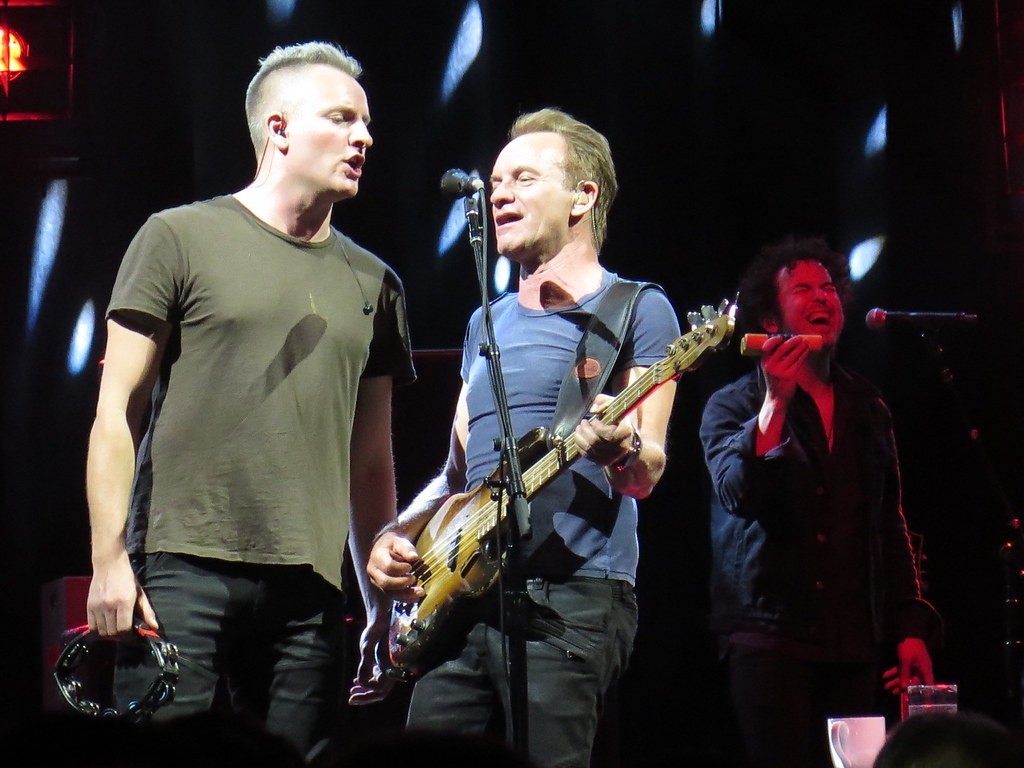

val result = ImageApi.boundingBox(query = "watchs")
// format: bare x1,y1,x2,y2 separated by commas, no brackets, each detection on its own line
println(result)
605,430,643,476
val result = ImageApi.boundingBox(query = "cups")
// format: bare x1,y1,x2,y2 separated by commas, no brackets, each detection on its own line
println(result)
827,716,885,768
908,686,958,716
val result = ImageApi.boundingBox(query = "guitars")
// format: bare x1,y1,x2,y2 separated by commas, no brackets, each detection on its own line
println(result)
384,294,742,672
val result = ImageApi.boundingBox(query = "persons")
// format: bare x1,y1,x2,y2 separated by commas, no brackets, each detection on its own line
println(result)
698,241,938,766
84,42,417,767
364,106,682,768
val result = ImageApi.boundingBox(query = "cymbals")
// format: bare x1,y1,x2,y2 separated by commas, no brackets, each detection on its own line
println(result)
55,618,182,732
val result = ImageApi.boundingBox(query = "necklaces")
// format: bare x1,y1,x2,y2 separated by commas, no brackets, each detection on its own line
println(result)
826,412,834,445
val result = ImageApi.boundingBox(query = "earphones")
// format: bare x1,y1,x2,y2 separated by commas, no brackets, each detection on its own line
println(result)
576,191,589,205
274,120,287,136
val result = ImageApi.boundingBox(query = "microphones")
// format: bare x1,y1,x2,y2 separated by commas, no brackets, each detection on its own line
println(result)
440,169,485,198
866,308,977,330
